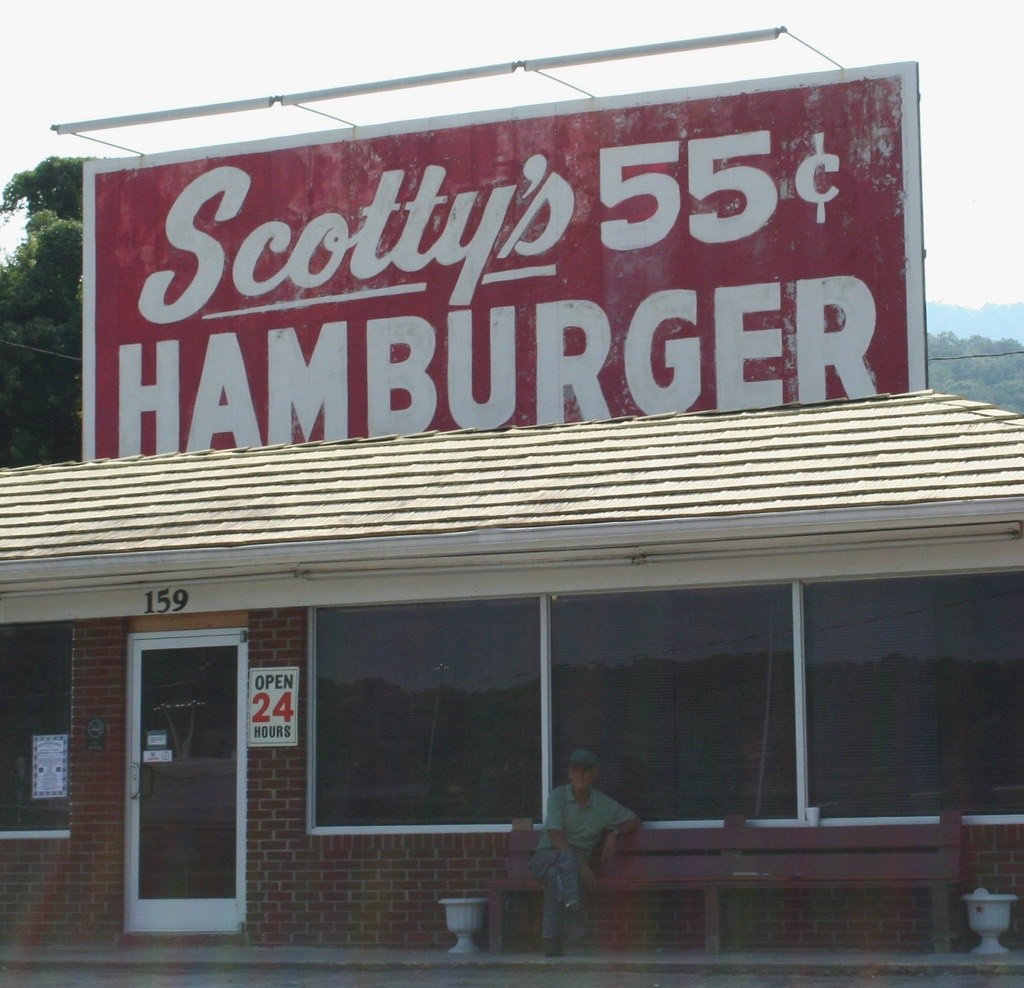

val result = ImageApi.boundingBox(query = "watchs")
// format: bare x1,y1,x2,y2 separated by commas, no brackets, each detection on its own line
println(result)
610,830,621,837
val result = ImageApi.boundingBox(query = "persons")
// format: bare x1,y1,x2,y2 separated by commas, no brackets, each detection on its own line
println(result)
530,746,643,958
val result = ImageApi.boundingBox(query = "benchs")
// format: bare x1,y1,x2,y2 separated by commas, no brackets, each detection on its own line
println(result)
486,816,976,951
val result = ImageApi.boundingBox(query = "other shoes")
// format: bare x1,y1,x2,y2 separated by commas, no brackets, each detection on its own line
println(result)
545,940,562,955
577,924,592,942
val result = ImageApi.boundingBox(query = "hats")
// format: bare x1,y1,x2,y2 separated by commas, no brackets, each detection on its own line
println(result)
569,750,600,766
